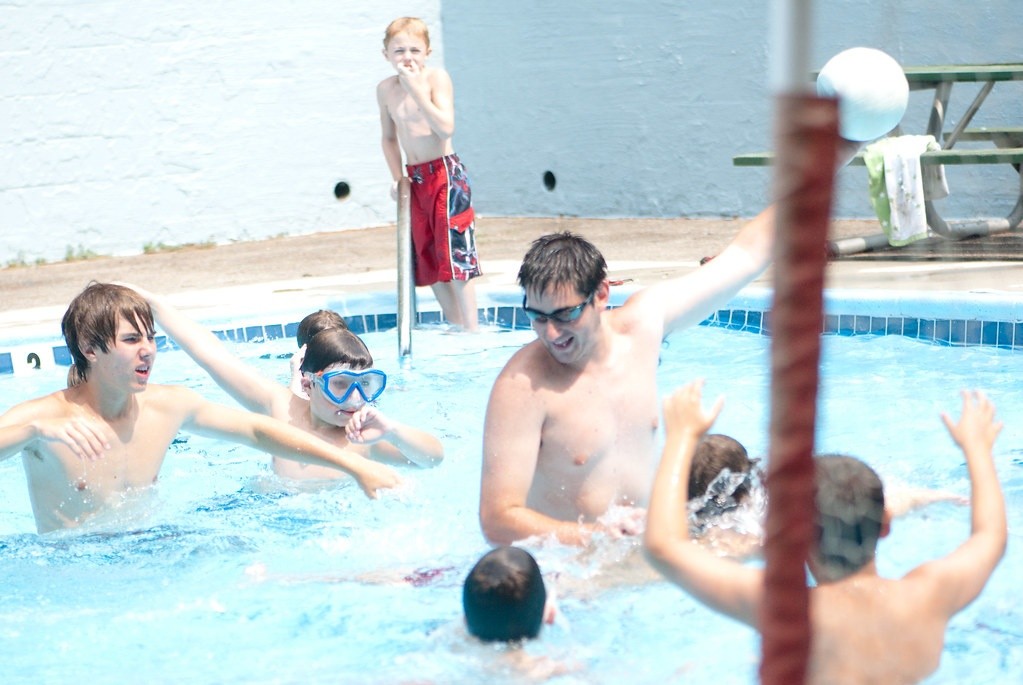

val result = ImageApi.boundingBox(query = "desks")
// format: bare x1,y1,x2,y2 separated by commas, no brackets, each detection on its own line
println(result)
812,63,1023,255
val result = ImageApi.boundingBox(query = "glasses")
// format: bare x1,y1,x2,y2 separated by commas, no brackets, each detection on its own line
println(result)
318,369,387,406
523,287,597,323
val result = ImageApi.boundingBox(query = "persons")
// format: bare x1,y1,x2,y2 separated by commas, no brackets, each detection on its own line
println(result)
479,140,868,549
463,545,547,641
687,435,751,518
111,280,444,481
644,380,1008,684
0,284,397,534
289,310,348,399
377,17,483,330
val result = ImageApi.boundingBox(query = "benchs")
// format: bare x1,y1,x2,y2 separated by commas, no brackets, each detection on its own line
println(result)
731,146,1023,167
944,127,1023,141
